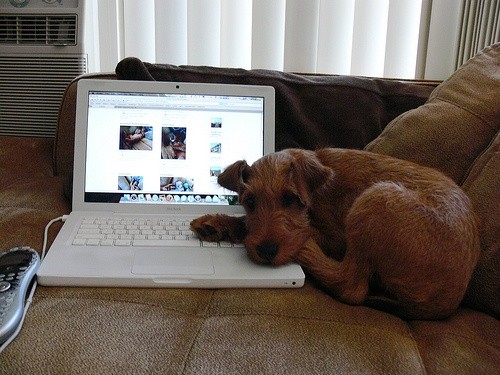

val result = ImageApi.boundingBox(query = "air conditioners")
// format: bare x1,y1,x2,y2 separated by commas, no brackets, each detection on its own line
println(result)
0,0,95,139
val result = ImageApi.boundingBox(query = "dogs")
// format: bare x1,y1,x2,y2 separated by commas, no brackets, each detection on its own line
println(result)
188,148,481,319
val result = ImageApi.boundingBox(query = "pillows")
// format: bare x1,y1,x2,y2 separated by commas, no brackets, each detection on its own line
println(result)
365,44,500,319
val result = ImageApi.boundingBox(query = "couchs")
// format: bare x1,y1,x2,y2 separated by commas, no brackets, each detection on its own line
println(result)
0,71,500,375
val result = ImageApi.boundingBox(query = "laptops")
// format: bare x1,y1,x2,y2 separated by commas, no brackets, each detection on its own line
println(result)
37,76,305,289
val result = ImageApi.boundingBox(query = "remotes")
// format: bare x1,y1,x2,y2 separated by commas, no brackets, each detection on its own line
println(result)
0,245,41,346
